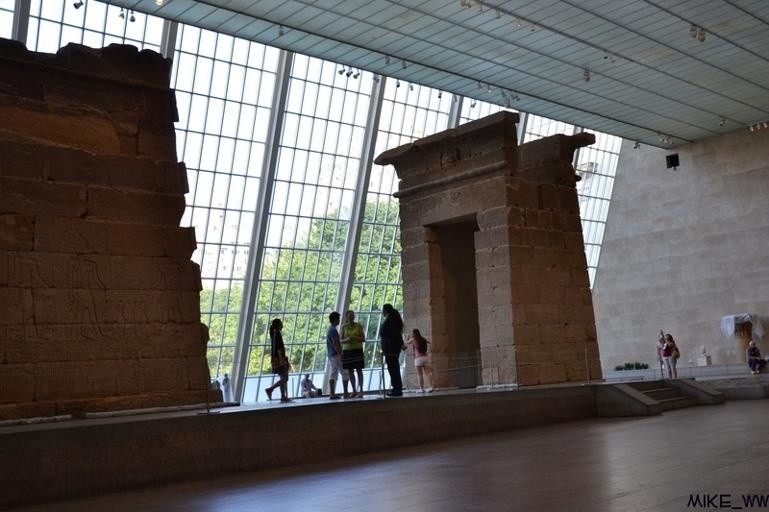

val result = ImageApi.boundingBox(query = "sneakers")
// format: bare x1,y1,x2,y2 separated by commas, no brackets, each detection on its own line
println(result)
265,388,294,403
750,369,761,375
328,390,362,399
416,386,436,395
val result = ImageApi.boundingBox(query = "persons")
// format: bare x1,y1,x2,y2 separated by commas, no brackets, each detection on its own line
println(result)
656,329,677,379
265,304,434,403
746,341,764,375
212,374,231,402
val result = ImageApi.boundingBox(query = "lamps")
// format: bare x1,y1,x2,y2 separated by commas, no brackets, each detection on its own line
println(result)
118,7,136,23
73,0,83,8
338,64,360,79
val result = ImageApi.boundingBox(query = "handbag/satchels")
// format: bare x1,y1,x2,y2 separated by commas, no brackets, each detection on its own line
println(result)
672,348,680,359
